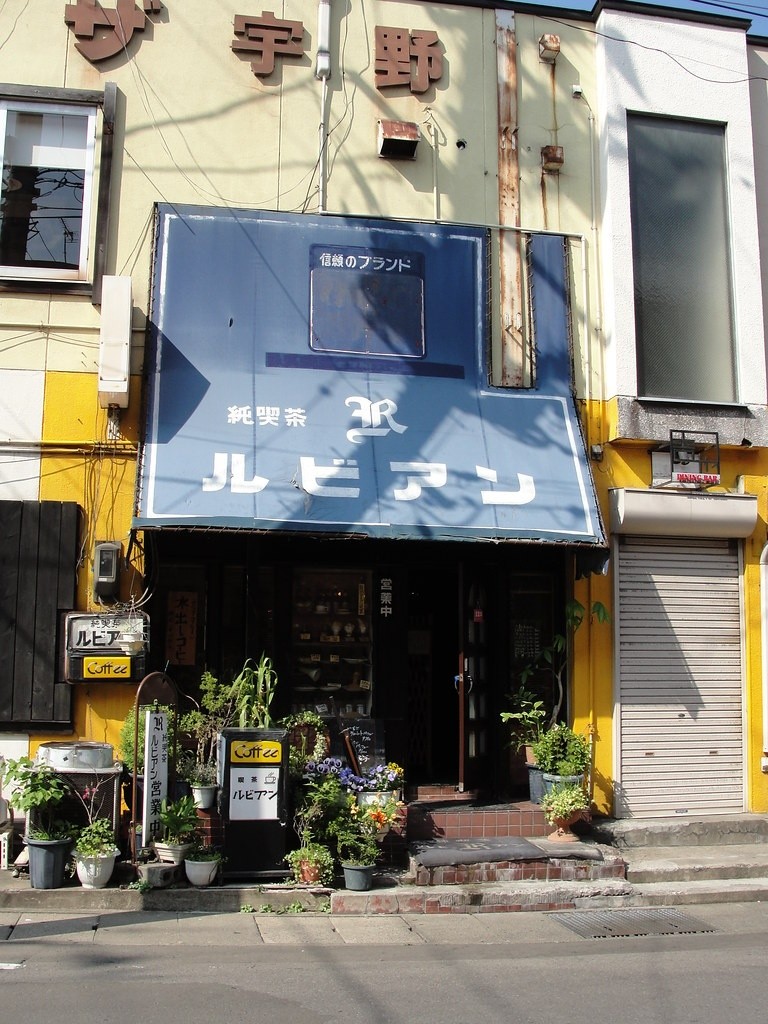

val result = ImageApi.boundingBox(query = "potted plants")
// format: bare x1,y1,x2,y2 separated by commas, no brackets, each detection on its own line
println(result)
3,724,405,896
502,686,595,842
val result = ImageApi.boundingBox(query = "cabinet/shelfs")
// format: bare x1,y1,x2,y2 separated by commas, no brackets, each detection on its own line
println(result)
296,601,370,719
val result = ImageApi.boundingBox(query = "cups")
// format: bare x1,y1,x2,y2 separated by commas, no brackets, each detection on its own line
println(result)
316,605,328,611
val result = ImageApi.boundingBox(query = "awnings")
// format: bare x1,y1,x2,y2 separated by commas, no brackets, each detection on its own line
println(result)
129,375,610,577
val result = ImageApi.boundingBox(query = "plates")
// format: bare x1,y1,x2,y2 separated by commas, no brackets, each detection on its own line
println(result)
342,687,363,691
320,686,340,692
342,657,368,664
291,686,318,691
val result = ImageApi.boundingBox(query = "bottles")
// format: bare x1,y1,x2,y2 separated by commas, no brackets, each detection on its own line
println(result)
343,587,349,608
333,586,340,611
